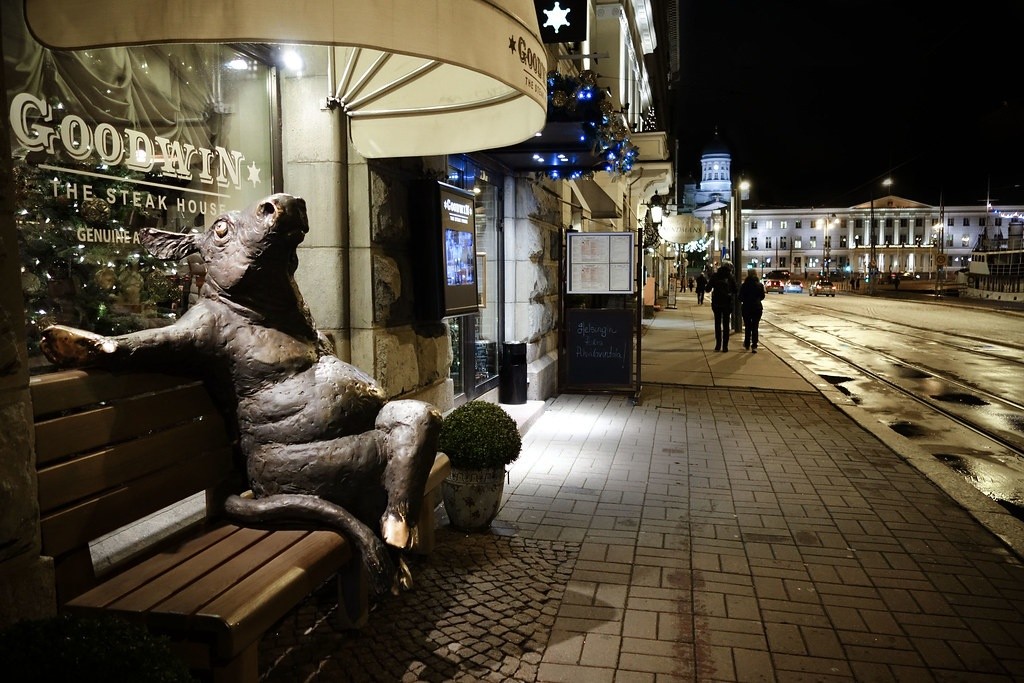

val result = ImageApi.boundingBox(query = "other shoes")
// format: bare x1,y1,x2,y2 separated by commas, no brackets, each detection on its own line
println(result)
752,348,758,353
715,345,721,350
723,346,728,352
743,343,750,350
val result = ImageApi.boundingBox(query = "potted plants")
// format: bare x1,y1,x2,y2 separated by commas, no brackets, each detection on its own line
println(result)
431,400,522,533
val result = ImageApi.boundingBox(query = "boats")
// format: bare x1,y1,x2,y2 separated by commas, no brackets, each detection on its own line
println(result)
953,173,1024,303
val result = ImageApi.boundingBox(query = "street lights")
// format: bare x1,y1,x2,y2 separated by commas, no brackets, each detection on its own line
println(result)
815,212,841,281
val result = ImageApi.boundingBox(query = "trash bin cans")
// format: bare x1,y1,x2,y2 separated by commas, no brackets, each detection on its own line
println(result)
499,341,528,404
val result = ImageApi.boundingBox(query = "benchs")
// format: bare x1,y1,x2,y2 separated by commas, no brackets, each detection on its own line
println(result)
26,329,452,681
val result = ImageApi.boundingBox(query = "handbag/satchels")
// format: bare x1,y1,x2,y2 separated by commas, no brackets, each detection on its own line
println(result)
720,279,744,301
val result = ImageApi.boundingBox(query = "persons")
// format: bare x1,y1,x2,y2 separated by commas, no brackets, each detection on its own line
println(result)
804,267,808,283
688,275,693,292
705,260,737,353
739,267,766,352
680,274,686,294
851,276,861,290
887,273,892,285
696,272,707,305
894,275,900,293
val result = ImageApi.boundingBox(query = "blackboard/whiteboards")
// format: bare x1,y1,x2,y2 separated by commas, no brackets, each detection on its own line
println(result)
564,307,633,388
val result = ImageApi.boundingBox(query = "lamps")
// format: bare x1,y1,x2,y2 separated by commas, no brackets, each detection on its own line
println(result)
568,47,598,64
596,86,611,98
612,103,630,113
629,123,637,129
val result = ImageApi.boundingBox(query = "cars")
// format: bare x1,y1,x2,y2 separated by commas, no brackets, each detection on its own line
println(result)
808,279,836,297
759,269,804,294
